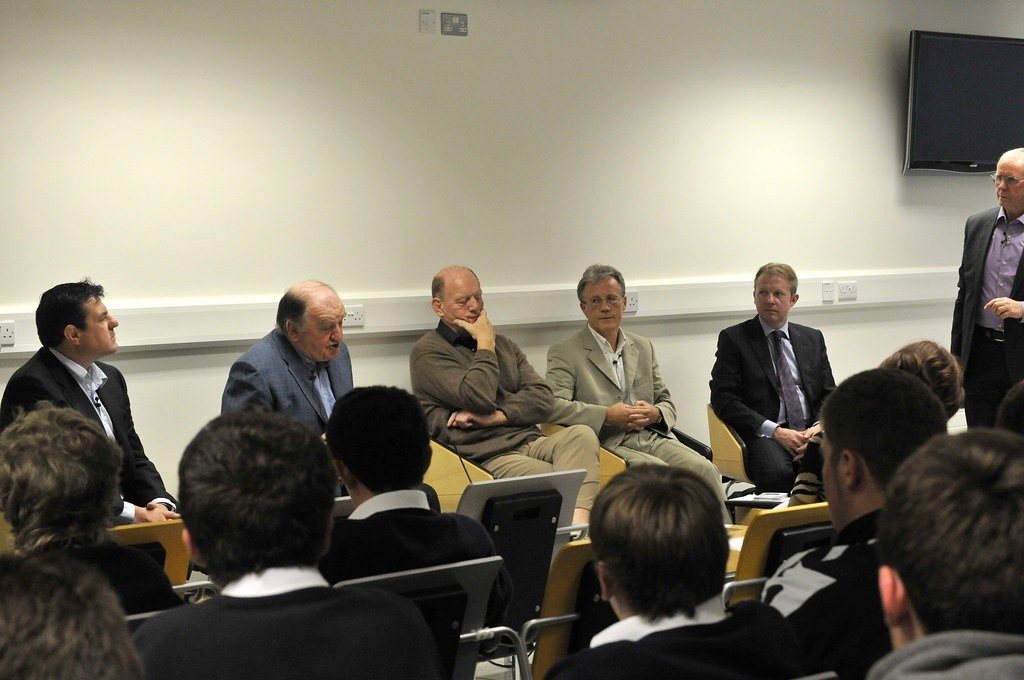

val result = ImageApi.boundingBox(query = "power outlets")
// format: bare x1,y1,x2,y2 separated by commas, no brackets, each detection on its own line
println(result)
421,10,436,35
0,320,16,346
839,282,858,299
350,305,365,327
624,291,639,313
441,13,469,37
822,282,834,302
342,304,350,327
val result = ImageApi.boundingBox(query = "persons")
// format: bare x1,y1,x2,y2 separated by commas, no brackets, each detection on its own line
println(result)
0,553,145,680
710,263,837,492
0,408,184,614
544,465,803,680
546,263,732,523
760,340,1024,680
132,416,441,679
950,148,1024,426
0,283,182,528
318,384,514,626
410,266,600,540
221,281,441,512
868,430,1024,680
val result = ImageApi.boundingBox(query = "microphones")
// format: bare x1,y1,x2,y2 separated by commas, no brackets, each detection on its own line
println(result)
95,397,99,401
613,360,617,364
310,370,314,375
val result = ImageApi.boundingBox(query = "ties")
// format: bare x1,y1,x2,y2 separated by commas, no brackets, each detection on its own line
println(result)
772,330,806,432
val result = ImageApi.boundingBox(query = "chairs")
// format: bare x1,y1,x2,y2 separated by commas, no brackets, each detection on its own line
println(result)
0,403,833,680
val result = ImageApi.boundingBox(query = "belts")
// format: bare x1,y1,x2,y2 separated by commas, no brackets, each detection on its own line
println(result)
975,324,1004,343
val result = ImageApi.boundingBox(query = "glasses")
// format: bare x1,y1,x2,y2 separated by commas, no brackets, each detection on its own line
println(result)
991,174,1024,185
581,294,624,309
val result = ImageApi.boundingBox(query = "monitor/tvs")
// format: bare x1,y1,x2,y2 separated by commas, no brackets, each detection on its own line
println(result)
902,29,1024,175
